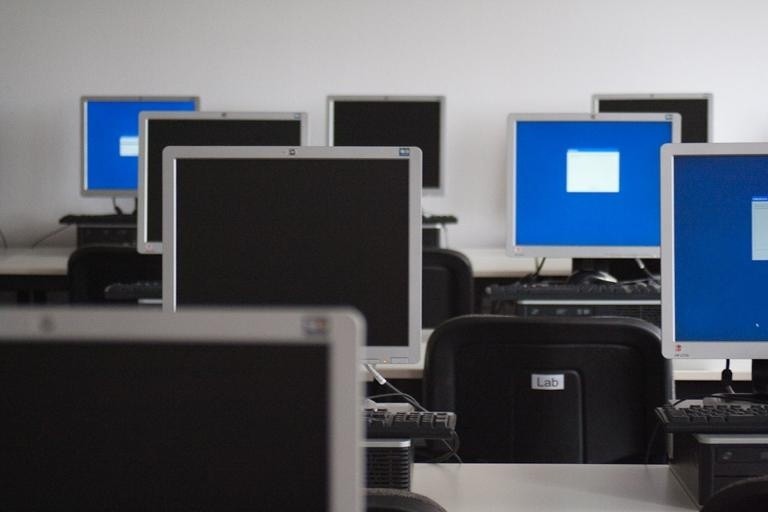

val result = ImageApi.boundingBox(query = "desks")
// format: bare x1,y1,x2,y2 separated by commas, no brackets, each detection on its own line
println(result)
441,243,574,278
1,241,72,275
362,335,753,385
408,460,702,512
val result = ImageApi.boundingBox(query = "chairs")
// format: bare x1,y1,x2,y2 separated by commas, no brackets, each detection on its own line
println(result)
423,314,676,464
421,246,474,315
65,247,166,306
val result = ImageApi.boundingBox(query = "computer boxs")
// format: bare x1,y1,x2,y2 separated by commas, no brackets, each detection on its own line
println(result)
694,438,768,512
422,226,441,248
76,224,138,247
67,241,163,304
571,257,659,283
517,299,659,326
358,445,415,491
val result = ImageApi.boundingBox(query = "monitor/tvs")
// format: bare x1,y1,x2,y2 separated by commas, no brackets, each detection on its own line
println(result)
505,112,678,284
660,141,768,399
77,94,200,221
137,110,312,257
0,303,371,512
328,91,450,196
590,91,716,146
159,141,430,382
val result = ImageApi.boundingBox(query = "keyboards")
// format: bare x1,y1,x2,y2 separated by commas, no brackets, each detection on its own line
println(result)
103,279,162,299
422,214,459,225
365,405,458,441
486,279,659,301
654,396,766,433
59,214,134,223
